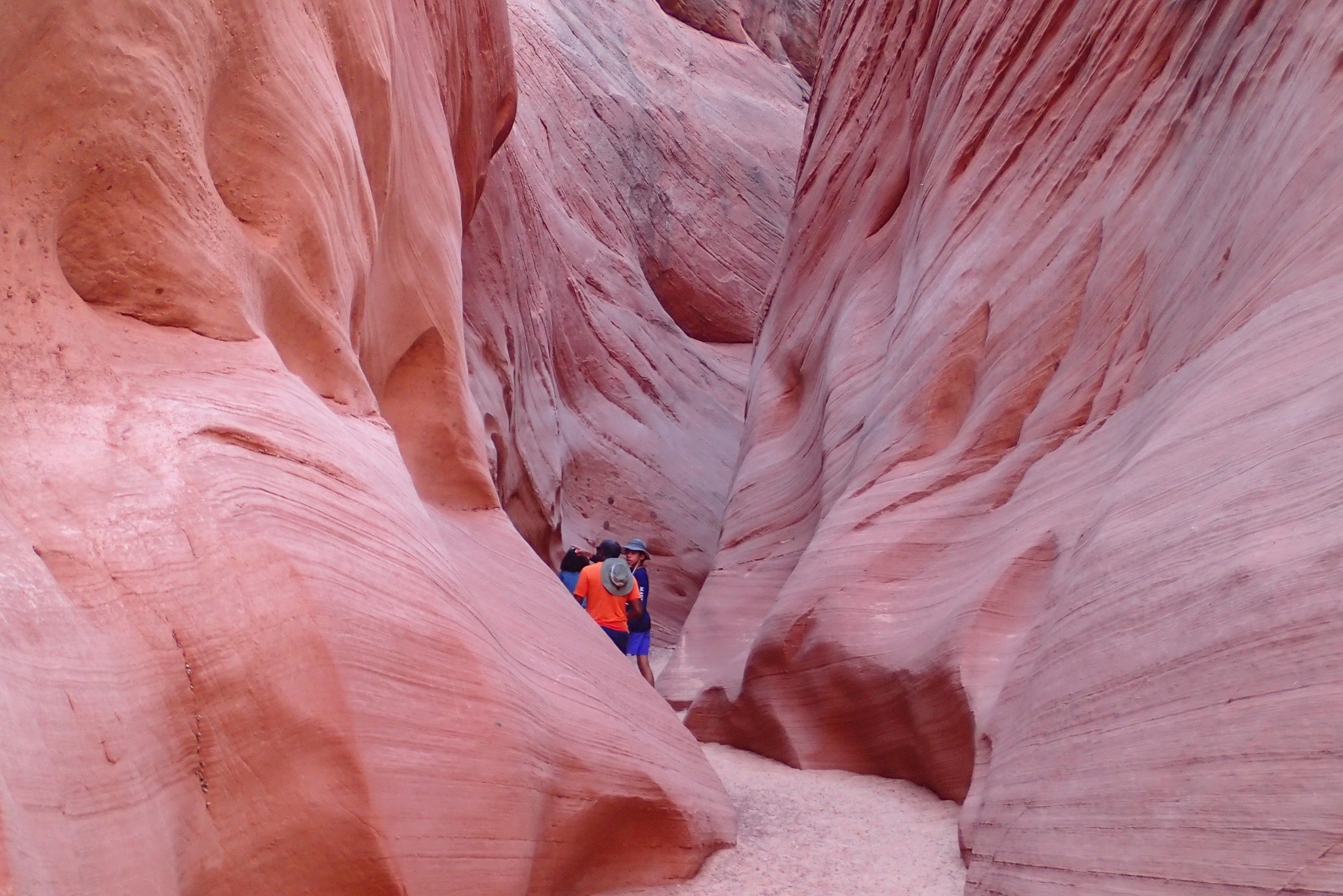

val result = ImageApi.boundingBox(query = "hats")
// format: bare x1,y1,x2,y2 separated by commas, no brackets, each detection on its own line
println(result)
600,557,633,596
620,538,651,560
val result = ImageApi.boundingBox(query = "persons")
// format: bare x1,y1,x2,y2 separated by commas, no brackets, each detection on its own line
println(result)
573,539,643,655
571,538,654,688
559,546,590,609
